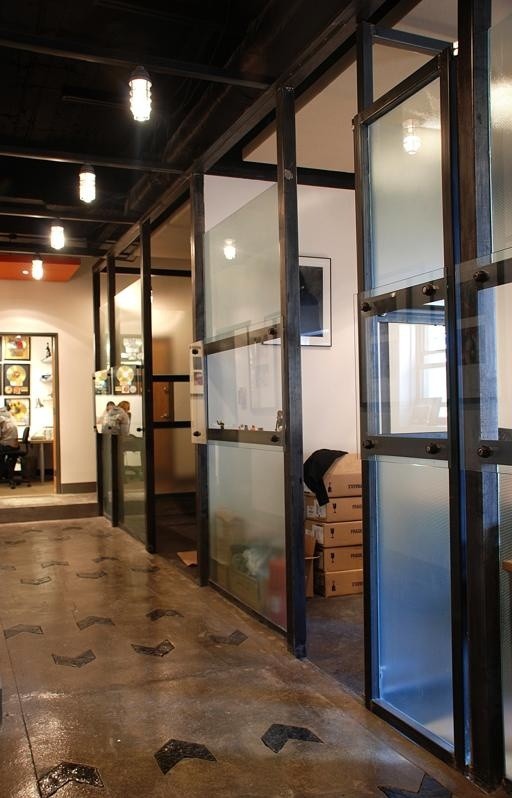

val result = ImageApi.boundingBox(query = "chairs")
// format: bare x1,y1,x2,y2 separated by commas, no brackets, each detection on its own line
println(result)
0,427,29,489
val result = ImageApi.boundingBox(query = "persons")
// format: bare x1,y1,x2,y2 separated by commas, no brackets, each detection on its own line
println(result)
104,400,131,433
1,406,20,483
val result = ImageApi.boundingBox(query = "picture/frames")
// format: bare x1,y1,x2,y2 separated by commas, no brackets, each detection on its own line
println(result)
0,334,31,427
261,256,332,347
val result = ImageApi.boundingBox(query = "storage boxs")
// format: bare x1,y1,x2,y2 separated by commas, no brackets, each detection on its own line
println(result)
209,453,363,629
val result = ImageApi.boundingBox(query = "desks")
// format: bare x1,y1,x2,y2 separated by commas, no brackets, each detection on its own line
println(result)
19,440,53,482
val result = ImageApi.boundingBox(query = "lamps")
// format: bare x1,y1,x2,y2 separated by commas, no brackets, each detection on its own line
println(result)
402,118,421,156
127,63,152,122
223,238,236,260
32,255,44,280
79,162,95,204
50,220,65,251
